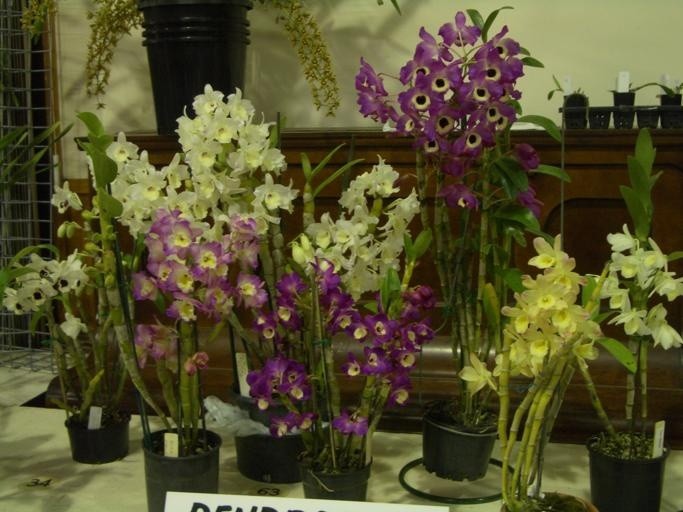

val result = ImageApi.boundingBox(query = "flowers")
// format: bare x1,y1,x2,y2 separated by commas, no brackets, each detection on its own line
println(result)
0,2,683,512
